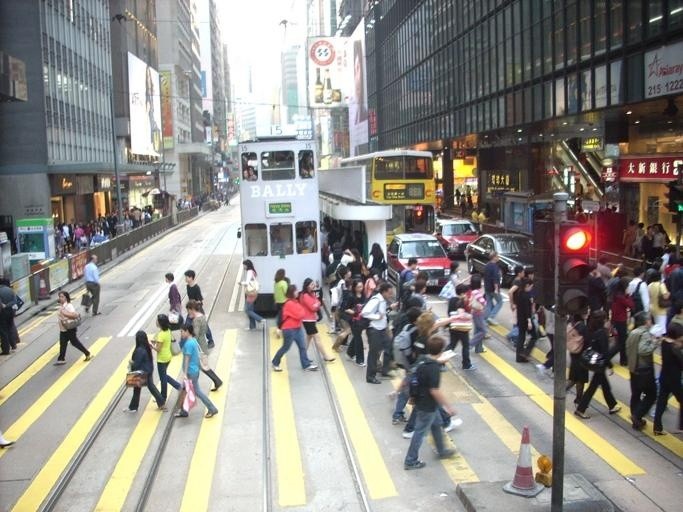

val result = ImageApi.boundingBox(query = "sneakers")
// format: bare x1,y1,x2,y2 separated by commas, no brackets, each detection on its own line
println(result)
84,354,91,362
53,360,67,365
336,335,397,385
272,356,333,371
386,389,463,469
537,364,667,437
123,381,223,418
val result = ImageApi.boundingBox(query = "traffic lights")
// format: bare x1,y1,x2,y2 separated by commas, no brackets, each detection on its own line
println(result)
559,224,593,320
662,180,678,213
667,183,682,227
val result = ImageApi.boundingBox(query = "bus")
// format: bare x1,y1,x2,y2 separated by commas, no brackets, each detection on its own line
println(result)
343,149,439,262
235,136,325,321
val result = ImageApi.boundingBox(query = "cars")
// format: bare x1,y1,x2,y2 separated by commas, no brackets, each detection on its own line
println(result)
388,232,450,294
465,233,534,286
437,219,478,256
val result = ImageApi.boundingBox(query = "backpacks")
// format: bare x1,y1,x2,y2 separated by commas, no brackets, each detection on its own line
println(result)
392,323,419,371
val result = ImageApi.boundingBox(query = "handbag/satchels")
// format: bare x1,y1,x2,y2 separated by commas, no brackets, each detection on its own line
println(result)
439,281,485,332
244,281,260,295
168,312,180,324
171,336,181,356
125,371,149,388
276,307,285,329
566,321,608,370
81,294,94,307
328,285,371,333
61,315,82,331
199,352,210,371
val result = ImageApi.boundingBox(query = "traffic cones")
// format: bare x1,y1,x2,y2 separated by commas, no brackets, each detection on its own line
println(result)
502,427,546,500
